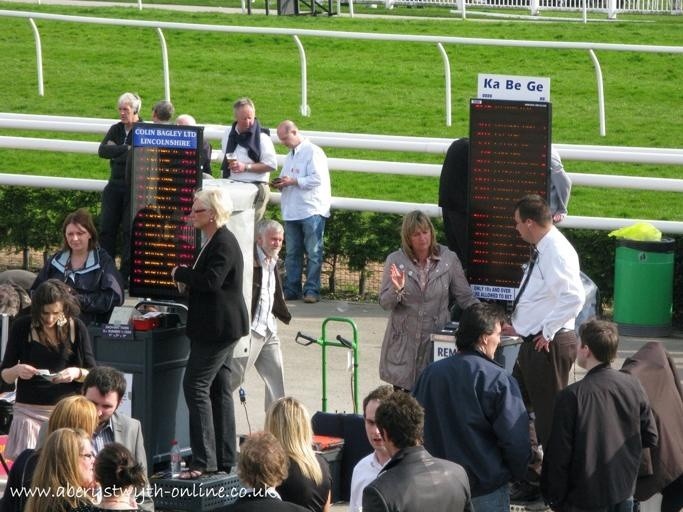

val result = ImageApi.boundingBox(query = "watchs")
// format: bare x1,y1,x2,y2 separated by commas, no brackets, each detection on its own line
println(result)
245,163,251,171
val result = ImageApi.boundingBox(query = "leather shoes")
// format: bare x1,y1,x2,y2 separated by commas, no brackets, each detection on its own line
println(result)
304,295,319,302
510,482,541,504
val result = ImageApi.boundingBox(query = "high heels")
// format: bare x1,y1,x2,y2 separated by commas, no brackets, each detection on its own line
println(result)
220,466,232,473
179,468,212,480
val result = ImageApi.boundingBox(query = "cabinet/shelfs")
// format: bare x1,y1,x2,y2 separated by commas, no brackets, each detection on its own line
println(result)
87,321,193,475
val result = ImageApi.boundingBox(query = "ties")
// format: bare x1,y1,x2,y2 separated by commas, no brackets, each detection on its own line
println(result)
512,249,537,313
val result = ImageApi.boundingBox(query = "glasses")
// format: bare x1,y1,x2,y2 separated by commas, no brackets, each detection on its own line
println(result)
191,206,207,214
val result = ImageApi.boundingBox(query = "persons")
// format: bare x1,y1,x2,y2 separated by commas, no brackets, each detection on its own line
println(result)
540,319,658,512
349,385,394,512
438,137,470,265
411,303,541,512
25,427,97,512
82,366,155,512
379,210,514,393
230,219,292,413
362,391,474,512
2,395,99,512
0,279,96,463
221,96,277,220
171,186,250,480
510,194,587,511
175,113,211,174
26,208,125,330
210,431,314,512
266,396,332,512
549,146,572,223
68,443,150,512
98,91,144,287
268,119,332,303
151,100,175,125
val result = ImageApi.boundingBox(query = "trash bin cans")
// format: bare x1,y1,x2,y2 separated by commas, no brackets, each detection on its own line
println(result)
612,236,676,339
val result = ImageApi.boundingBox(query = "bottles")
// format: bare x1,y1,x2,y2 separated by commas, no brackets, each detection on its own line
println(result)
169,439,181,478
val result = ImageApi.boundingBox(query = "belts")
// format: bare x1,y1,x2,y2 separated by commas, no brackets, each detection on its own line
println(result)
523,329,568,343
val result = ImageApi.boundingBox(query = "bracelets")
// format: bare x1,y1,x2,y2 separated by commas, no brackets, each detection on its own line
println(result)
75,367,82,381
